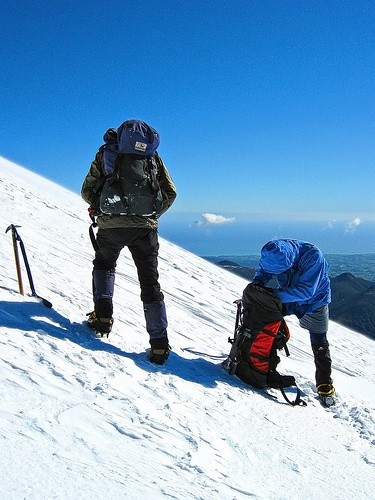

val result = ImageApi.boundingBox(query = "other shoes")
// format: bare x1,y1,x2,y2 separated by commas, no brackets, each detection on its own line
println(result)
145,344,176,366
83,309,114,340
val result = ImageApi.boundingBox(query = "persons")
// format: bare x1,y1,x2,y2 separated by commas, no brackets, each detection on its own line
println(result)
252,237,340,408
81,128,177,365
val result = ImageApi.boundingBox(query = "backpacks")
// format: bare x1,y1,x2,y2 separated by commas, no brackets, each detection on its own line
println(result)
227,284,302,405
102,118,164,220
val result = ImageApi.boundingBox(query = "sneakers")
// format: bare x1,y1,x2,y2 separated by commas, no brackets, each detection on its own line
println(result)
313,376,338,408
